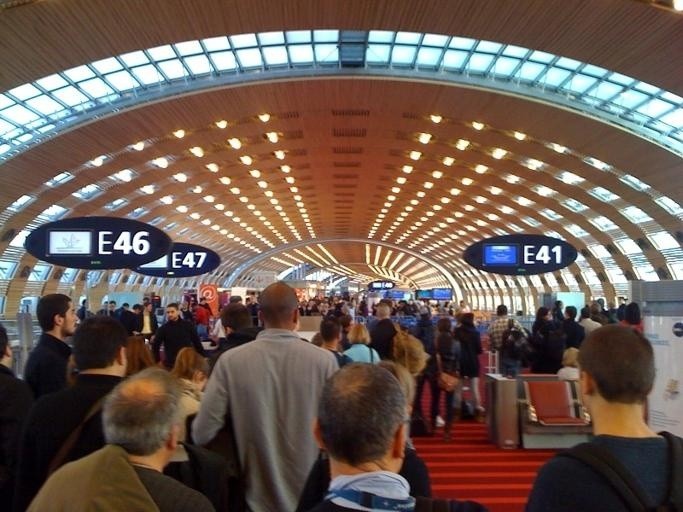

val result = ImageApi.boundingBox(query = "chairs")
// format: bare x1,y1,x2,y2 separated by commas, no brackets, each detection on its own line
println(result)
523,380,590,449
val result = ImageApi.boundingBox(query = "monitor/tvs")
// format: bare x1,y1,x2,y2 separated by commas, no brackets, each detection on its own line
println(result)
46,227,94,257
140,253,169,270
384,288,452,300
482,242,521,268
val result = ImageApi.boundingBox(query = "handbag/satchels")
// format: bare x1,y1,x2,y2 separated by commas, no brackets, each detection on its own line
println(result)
438,372,460,392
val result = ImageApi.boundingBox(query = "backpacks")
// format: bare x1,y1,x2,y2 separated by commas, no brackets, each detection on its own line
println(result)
502,319,533,358
392,322,426,377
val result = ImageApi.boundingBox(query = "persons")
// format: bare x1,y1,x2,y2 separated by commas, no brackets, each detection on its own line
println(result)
2,280,491,512
485,294,646,376
524,324,682,510
